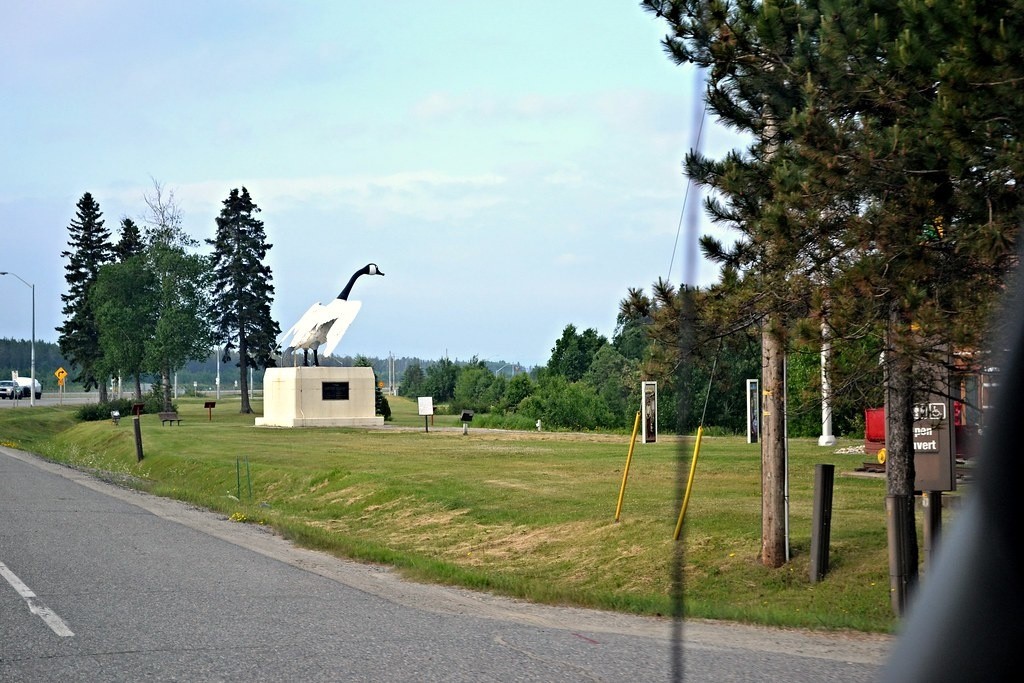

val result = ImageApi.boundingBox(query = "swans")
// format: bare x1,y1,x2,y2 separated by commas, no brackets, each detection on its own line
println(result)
267,263,385,367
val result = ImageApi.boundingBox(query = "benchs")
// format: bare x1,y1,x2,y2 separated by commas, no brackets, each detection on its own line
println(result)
158,412,183,426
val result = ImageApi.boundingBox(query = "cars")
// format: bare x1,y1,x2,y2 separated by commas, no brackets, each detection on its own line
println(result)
0,380,22,399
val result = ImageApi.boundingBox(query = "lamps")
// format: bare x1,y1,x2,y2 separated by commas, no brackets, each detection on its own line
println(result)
460,409,474,423
111,411,121,419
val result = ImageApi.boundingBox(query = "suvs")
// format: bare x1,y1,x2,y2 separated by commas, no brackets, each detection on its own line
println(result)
16,377,41,399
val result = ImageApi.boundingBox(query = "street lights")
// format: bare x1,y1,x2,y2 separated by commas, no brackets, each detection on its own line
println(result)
0,271,35,406
